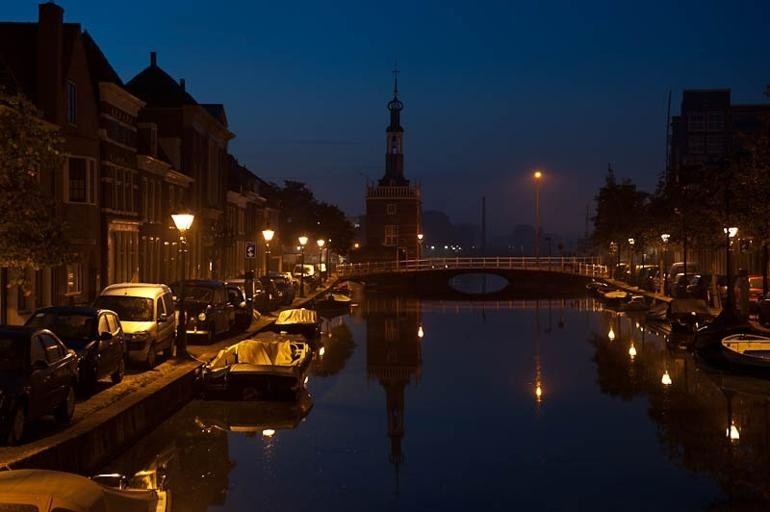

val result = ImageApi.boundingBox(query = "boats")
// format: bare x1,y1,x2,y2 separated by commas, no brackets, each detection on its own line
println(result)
586,279,632,303
0,458,173,511
323,288,355,306
720,332,770,372
716,364,770,402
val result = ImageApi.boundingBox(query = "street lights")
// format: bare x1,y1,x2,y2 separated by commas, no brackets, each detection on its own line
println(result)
599,304,741,448
317,239,325,287
722,224,740,327
169,212,196,358
416,274,428,340
262,228,276,313
531,271,547,411
416,232,424,269
627,237,637,286
355,241,360,272
298,235,309,297
661,232,669,296
533,169,543,265
608,240,615,279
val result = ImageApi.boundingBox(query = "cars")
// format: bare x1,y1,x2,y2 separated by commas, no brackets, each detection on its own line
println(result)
615,262,770,319
